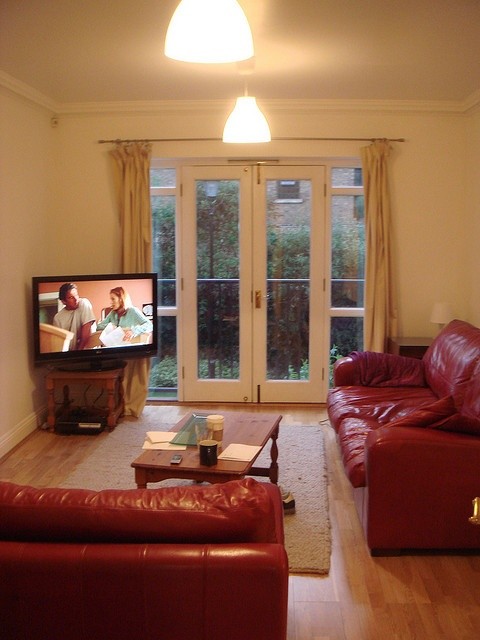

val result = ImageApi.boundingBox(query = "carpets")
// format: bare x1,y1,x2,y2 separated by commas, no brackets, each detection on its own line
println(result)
56,419,335,581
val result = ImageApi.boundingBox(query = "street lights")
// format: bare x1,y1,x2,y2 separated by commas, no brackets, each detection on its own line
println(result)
206,183,218,345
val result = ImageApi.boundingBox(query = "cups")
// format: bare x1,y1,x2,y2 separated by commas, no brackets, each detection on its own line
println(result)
195,422,213,451
199,440,218,468
206,415,224,448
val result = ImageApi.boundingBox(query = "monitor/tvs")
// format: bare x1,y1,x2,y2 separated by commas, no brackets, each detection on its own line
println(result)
32,272,158,372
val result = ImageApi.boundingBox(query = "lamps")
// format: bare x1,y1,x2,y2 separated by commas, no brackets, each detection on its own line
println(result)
163,0,255,65
222,71,272,145
431,302,454,328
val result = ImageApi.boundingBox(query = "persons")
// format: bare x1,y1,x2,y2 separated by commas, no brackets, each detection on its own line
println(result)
96,287,152,342
53,282,101,351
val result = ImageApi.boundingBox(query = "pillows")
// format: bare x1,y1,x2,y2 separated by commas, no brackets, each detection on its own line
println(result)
428,412,479,435
377,394,459,429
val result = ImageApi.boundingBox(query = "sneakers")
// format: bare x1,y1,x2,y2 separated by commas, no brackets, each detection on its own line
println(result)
282,492,295,514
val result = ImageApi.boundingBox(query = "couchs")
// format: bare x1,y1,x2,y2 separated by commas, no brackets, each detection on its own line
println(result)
324,318,479,552
1,477,291,638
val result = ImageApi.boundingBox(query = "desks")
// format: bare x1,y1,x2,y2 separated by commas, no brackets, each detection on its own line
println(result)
44,364,128,433
388,335,438,359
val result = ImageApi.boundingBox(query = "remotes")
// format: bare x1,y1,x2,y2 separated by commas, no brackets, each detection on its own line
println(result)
170,454,183,465
